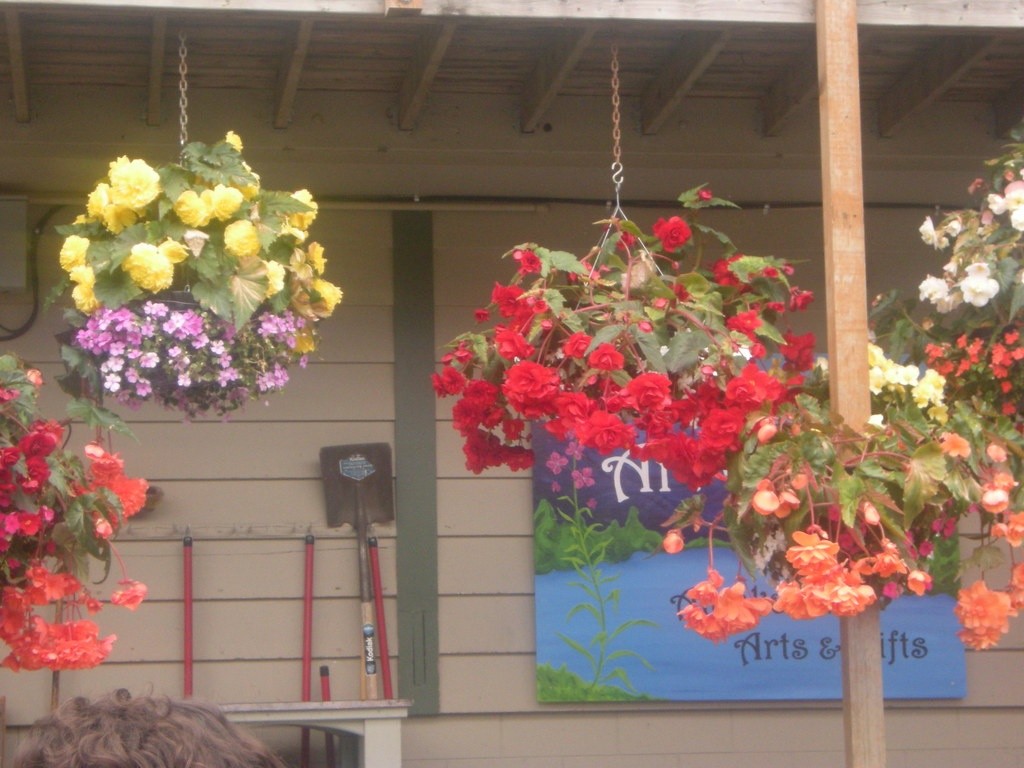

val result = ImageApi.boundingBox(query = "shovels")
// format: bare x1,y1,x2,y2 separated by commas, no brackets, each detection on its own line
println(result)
319,441,395,701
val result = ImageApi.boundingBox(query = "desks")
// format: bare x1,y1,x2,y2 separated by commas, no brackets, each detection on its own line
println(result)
218,698,412,768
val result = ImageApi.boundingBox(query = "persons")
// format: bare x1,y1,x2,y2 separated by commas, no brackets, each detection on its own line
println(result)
0,687,291,768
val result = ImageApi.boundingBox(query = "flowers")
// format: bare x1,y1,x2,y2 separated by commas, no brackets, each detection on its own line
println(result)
431,130,1023,651
0,351,151,673
44,130,344,429
